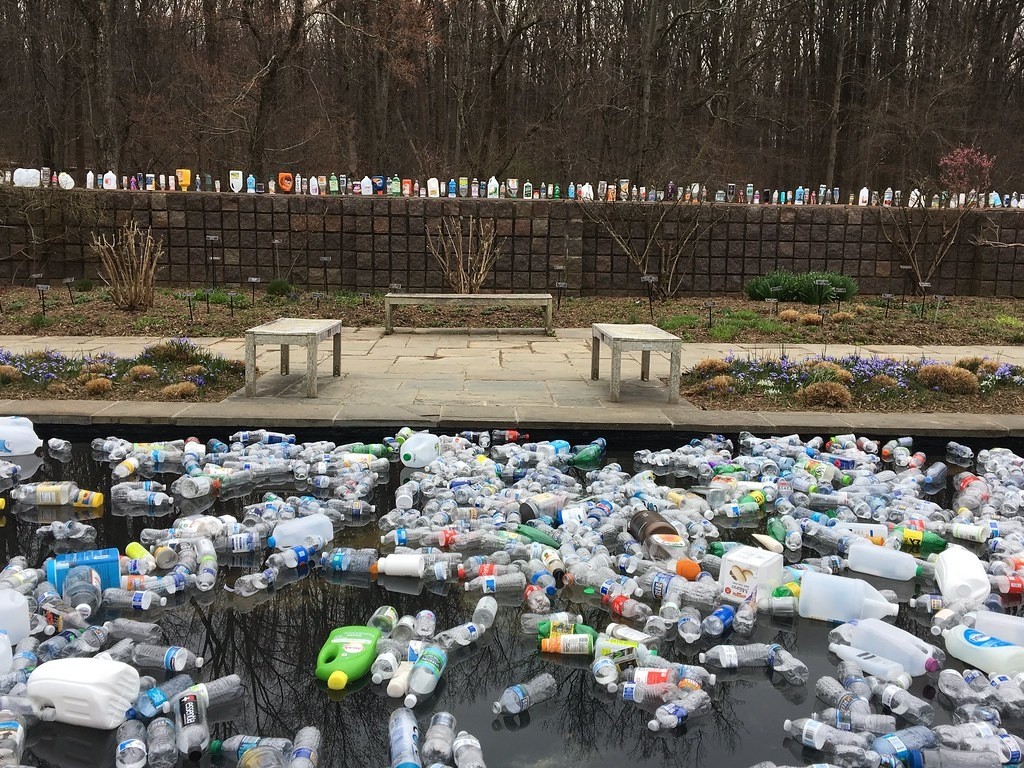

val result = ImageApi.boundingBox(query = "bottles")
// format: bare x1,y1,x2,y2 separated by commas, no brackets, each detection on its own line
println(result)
123,176,127,190
568,182,593,201
353,172,426,197
989,190,1024,209
160,175,166,190
13,168,40,187
130,176,136,190
598,179,664,203
52,171,57,187
40,167,50,188
86,171,94,190
146,174,155,190
195,174,201,191
427,175,518,199
176,169,190,191
0,414,1024,768
0,170,4,185
5,171,11,186
103,171,116,189
169,176,175,191
848,186,901,207
763,184,839,206
58,170,74,190
97,174,103,189
279,173,352,195
667,181,706,203
205,170,276,194
522,179,560,199
715,183,760,204
137,173,144,190
908,188,985,209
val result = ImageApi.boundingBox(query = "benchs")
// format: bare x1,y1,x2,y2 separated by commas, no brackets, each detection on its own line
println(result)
384,293,553,336
245,317,342,398
591,324,682,405
